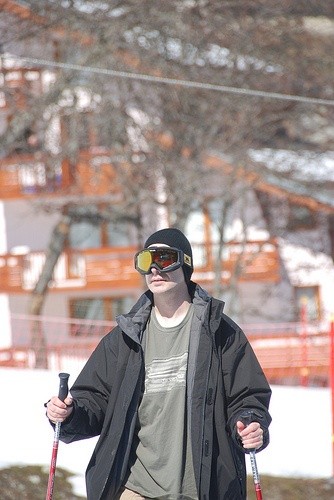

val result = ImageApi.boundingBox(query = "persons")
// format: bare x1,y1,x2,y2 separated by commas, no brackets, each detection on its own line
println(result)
45,228,272,500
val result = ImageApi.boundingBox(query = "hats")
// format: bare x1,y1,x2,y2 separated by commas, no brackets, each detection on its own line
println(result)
144,228,193,272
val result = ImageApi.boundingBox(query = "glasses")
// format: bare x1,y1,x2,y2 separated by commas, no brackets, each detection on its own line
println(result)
134,246,184,275
